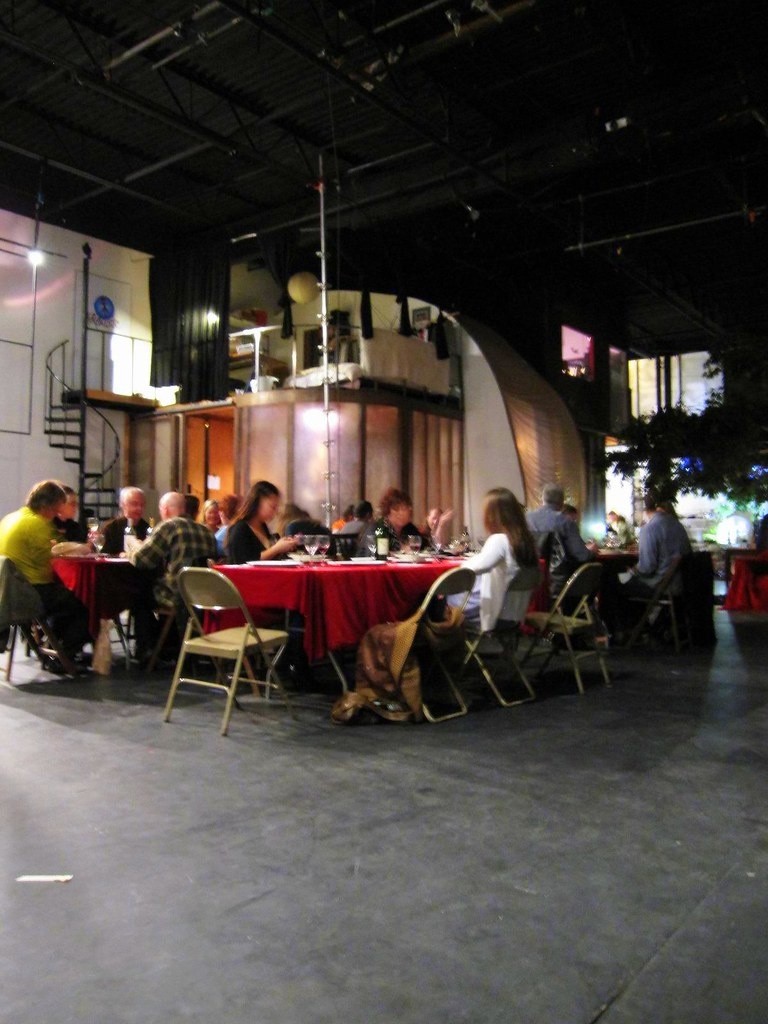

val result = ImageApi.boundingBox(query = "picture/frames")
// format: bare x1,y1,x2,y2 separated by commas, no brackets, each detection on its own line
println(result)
76,269,133,335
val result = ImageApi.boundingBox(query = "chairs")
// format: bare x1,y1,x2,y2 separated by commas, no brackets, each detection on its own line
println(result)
0,557,81,682
162,566,290,737
421,550,683,725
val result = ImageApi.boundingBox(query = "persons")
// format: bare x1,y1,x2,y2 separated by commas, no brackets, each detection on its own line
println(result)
750,515,768,548
0,480,535,708
601,492,747,646
527,485,650,647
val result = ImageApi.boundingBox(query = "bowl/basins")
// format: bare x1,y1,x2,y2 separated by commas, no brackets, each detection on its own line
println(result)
287,554,327,562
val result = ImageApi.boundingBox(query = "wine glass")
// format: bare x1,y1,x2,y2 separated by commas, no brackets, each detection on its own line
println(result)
316,534,330,567
408,535,421,565
293,534,307,568
366,535,376,558
92,531,104,559
87,516,99,541
304,535,318,568
431,532,472,556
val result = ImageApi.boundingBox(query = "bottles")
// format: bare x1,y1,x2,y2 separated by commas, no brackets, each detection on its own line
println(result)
374,516,389,559
124,517,137,552
146,517,156,539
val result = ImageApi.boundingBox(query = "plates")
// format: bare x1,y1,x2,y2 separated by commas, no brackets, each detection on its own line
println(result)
95,556,129,562
436,555,465,560
350,557,375,560
327,560,386,565
386,556,434,562
393,553,427,558
60,553,108,557
246,561,302,566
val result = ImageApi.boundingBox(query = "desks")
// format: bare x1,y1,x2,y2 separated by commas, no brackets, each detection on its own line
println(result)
51,555,133,670
204,556,468,699
